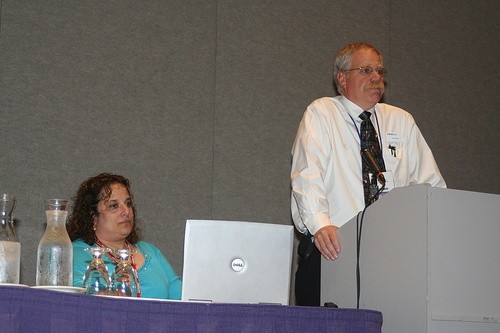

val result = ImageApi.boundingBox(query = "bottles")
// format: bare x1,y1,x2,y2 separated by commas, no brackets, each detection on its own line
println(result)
36,199,73,288
0,192,21,285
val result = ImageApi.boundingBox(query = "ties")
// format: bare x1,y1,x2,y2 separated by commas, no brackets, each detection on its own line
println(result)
358,110,385,207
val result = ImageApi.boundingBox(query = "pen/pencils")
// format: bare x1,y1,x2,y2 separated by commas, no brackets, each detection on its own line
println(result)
389,145,396,157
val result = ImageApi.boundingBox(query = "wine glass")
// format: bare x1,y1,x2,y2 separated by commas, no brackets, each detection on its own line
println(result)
82,248,112,296
110,249,139,298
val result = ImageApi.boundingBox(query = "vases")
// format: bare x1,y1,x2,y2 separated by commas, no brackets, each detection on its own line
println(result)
0,193,20,285
36,199,73,288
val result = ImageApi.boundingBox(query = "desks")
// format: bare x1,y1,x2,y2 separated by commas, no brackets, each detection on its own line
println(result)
0,284,383,333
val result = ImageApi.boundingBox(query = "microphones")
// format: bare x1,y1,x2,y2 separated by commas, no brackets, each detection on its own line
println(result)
361,146,385,182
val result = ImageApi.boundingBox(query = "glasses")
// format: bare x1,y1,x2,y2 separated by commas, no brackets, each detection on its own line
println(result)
342,66,389,76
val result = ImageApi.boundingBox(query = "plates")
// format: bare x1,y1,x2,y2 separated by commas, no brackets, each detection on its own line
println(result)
30,285,85,293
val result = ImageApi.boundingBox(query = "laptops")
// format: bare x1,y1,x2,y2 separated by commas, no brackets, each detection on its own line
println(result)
181,219,294,306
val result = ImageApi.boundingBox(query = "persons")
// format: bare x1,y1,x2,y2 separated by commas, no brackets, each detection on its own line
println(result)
290,43,447,307
66,173,182,300
